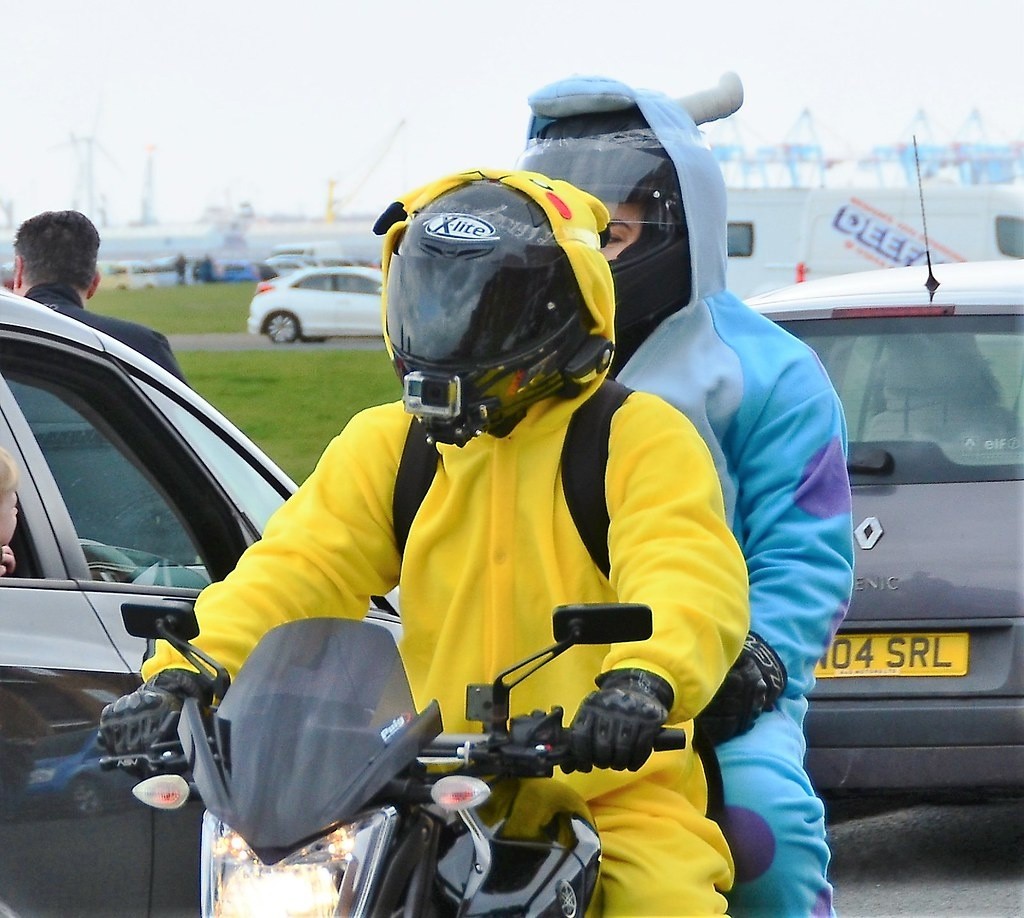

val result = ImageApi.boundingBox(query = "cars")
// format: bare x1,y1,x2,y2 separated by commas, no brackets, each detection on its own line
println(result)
726,258,1024,834
107,240,381,285
0,283,407,918
246,264,384,345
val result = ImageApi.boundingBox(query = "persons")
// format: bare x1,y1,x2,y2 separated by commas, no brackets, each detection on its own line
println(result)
0,445,20,576
97,165,750,918
521,75,854,918
13,210,190,385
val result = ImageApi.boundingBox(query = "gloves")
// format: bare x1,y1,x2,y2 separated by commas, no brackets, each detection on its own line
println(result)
560,668,674,775
99,669,214,780
691,630,787,753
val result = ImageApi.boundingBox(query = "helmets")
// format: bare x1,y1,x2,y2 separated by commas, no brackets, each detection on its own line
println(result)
385,179,615,448
522,107,692,337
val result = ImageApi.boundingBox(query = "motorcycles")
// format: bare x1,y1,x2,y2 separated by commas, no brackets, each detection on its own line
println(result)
91,597,685,918
